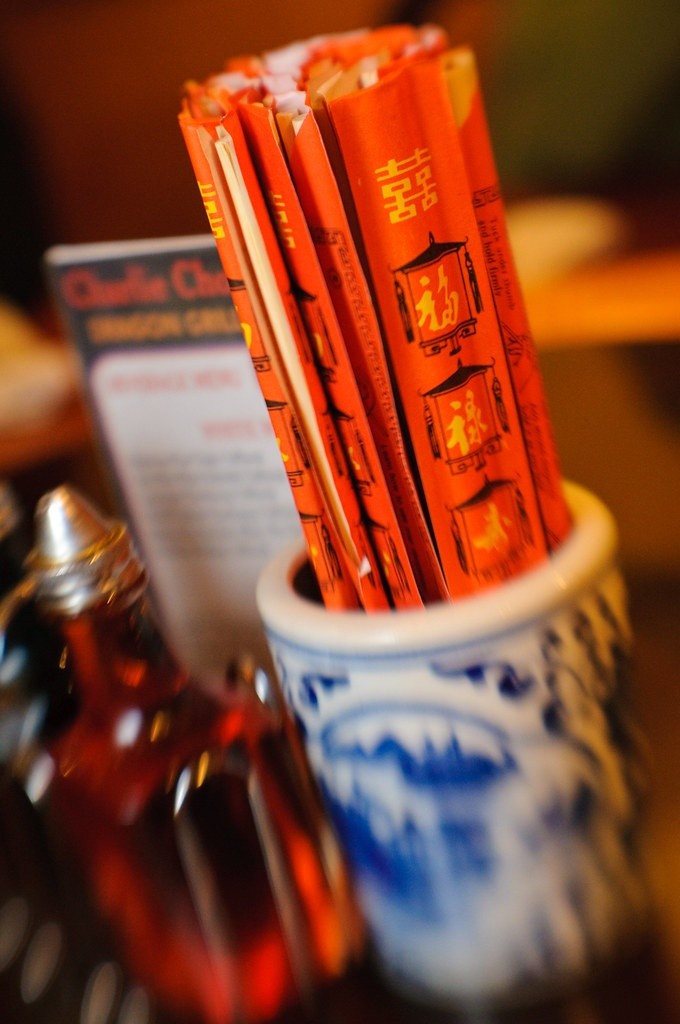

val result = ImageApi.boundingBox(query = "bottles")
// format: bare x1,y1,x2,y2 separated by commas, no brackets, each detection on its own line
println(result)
24,489,372,1019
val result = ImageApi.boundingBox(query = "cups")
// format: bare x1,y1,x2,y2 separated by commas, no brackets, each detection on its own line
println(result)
259,479,652,1015
